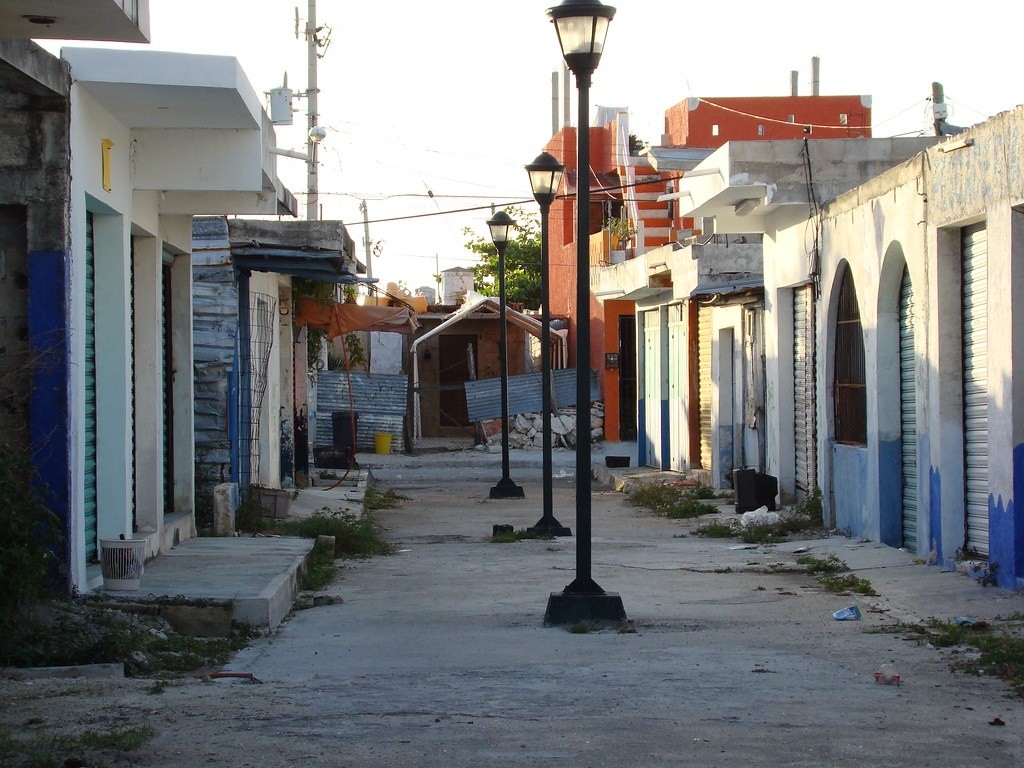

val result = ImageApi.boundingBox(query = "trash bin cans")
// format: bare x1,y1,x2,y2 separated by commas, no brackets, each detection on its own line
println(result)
330,411,359,453
375,432,393,454
99,536,146,592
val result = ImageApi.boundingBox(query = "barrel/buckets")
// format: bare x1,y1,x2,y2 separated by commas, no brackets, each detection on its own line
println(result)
330,411,359,454
374,433,393,454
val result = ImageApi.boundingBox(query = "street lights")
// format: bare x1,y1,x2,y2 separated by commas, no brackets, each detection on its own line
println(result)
526,151,574,540
486,211,526,500
544,1,630,627
